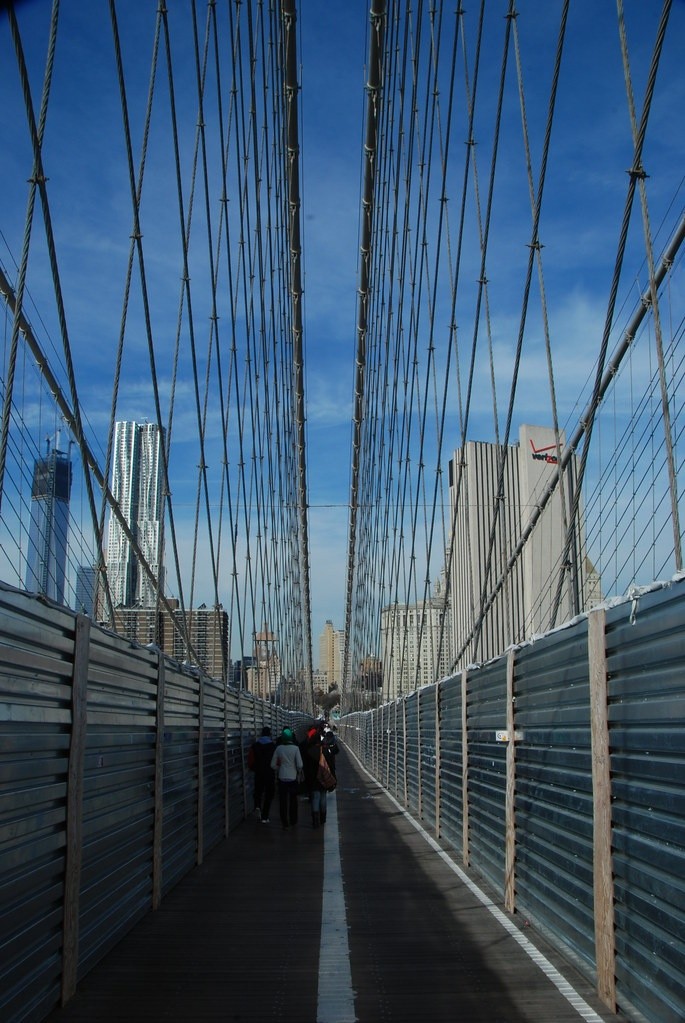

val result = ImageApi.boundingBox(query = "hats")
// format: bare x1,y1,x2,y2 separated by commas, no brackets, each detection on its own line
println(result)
308,729,315,737
282,729,292,740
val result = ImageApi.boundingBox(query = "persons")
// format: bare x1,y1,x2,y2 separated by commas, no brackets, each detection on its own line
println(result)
247,721,339,832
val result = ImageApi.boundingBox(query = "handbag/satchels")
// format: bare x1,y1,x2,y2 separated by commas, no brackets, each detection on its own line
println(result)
316,746,336,788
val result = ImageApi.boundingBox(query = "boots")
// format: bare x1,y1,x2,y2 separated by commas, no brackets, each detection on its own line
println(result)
321,811,326,824
312,811,320,828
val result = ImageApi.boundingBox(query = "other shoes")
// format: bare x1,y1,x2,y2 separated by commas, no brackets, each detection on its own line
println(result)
283,827,289,830
255,808,261,823
261,818,271,824
